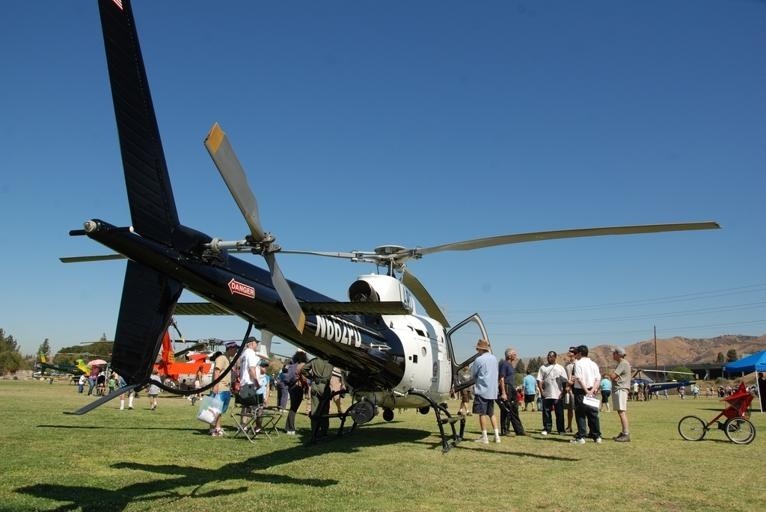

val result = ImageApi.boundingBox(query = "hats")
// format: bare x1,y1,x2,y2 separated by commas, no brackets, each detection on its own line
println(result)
476,339,490,352
257,359,269,366
244,337,260,343
610,347,626,356
225,342,238,349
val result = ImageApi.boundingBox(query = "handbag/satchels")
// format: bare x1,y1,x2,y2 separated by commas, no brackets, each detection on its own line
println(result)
195,392,225,426
234,384,257,406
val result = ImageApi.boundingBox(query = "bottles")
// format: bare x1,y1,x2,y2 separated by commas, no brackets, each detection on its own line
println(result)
564,390,571,405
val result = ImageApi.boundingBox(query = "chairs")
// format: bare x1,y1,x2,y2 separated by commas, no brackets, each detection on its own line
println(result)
230,384,290,444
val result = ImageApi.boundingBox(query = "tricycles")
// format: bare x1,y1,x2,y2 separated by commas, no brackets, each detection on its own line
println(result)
677,379,758,445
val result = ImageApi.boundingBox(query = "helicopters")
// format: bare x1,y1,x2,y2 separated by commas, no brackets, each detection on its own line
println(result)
628,367,700,399
32,334,173,385
49,0,725,451
73,313,299,395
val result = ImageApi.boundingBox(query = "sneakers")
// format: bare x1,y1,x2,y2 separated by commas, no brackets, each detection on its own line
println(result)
474,436,489,445
540,426,602,445
493,430,530,444
613,432,631,442
